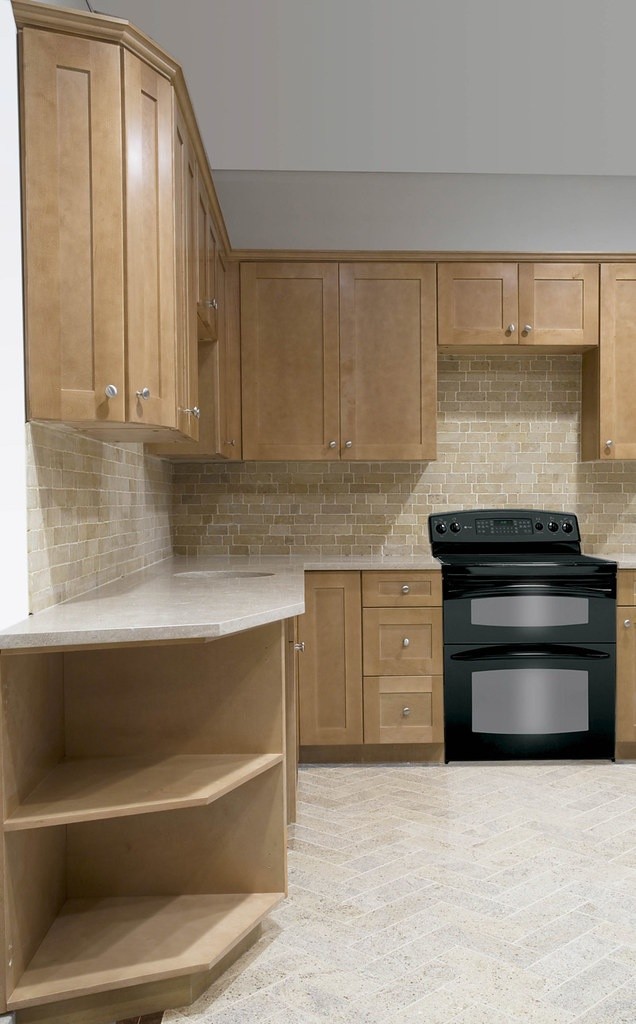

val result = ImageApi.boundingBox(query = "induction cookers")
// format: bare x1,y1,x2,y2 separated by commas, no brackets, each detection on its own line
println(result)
428,509,617,575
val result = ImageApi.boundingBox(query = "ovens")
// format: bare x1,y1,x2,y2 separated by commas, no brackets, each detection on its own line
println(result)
442,574,616,763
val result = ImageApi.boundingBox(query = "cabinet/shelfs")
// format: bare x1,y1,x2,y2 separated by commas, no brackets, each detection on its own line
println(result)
613,569,636,763
19,22,635,461
0,569,440,1024
